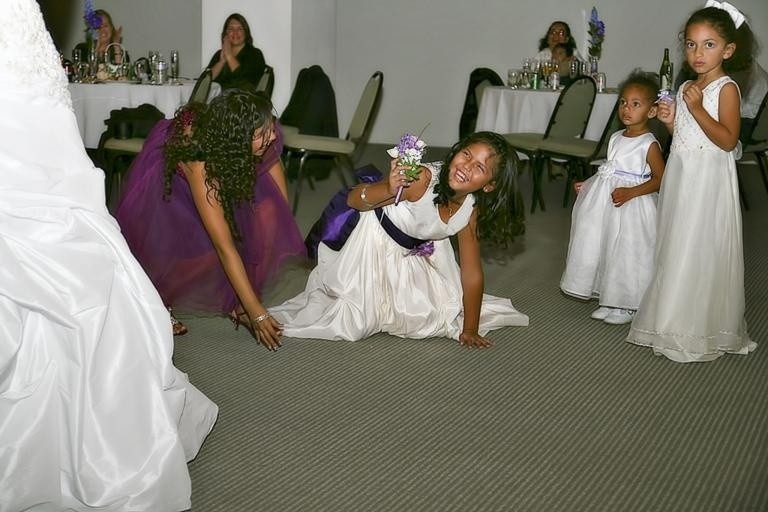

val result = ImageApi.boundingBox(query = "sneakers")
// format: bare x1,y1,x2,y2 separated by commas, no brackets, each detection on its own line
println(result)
590,306,634,325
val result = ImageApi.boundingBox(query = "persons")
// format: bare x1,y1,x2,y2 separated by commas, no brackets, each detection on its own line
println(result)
548,43,575,77
529,22,586,183
114,89,308,352
72,9,129,67
559,69,665,326
206,14,267,91
625,1,758,363
1,0,223,512
263,132,529,350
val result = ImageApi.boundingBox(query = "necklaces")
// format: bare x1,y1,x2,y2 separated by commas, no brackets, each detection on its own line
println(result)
443,192,457,217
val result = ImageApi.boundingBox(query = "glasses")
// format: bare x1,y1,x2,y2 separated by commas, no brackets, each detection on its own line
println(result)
549,30,567,36
226,27,244,32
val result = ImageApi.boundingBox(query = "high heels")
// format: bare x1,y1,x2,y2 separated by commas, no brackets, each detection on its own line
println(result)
229,310,282,338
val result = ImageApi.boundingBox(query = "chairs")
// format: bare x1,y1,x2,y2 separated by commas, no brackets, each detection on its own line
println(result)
736,93,768,215
503,75,598,214
470,67,504,114
278,66,335,189
255,64,275,101
530,71,660,216
284,70,385,217
102,71,214,207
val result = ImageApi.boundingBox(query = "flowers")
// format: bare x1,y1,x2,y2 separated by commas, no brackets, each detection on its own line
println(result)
587,7,605,58
81,0,103,30
387,119,436,207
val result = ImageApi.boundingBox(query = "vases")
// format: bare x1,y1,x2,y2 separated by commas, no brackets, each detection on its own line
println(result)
86,29,102,76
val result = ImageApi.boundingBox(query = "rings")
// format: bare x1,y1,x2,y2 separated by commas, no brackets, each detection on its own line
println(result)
258,330,263,336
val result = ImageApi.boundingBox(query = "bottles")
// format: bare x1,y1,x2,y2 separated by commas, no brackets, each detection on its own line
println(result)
507,58,607,93
660,48,673,93
60,48,180,85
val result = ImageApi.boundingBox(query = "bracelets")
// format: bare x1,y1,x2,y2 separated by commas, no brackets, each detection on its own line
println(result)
247,311,273,325
112,51,122,57
362,182,376,208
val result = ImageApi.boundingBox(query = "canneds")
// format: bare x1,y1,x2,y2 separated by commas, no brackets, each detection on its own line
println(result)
596,72,606,94
154,63,167,85
551,72,560,91
531,73,540,91
